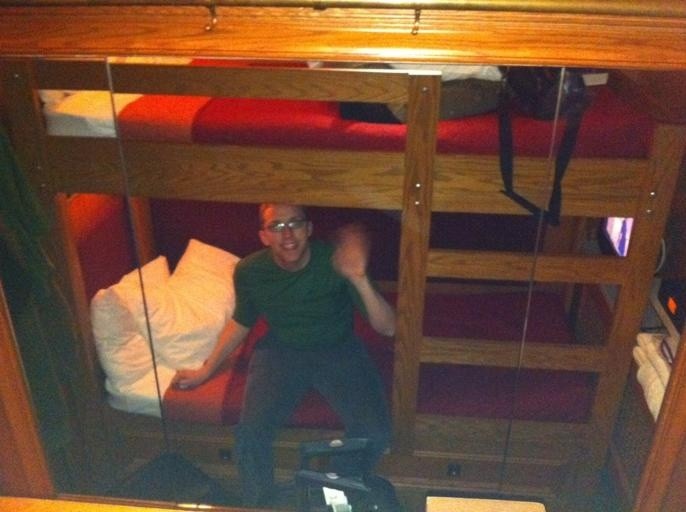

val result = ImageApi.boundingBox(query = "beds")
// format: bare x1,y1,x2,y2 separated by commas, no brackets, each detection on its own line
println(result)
0,7,686,510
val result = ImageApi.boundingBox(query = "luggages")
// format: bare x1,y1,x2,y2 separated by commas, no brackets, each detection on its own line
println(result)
260,437,402,512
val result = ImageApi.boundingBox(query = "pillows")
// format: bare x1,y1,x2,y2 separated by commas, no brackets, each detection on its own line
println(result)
90,236,247,389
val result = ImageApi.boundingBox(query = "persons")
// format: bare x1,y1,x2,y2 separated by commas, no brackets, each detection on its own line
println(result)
170,202,396,508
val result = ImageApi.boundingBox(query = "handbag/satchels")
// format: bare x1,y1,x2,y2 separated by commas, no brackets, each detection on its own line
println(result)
499,66,584,118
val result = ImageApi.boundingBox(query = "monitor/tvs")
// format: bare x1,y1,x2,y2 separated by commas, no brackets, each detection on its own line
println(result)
598,216,634,256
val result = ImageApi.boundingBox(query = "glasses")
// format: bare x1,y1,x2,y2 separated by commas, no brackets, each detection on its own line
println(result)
267,220,306,231
